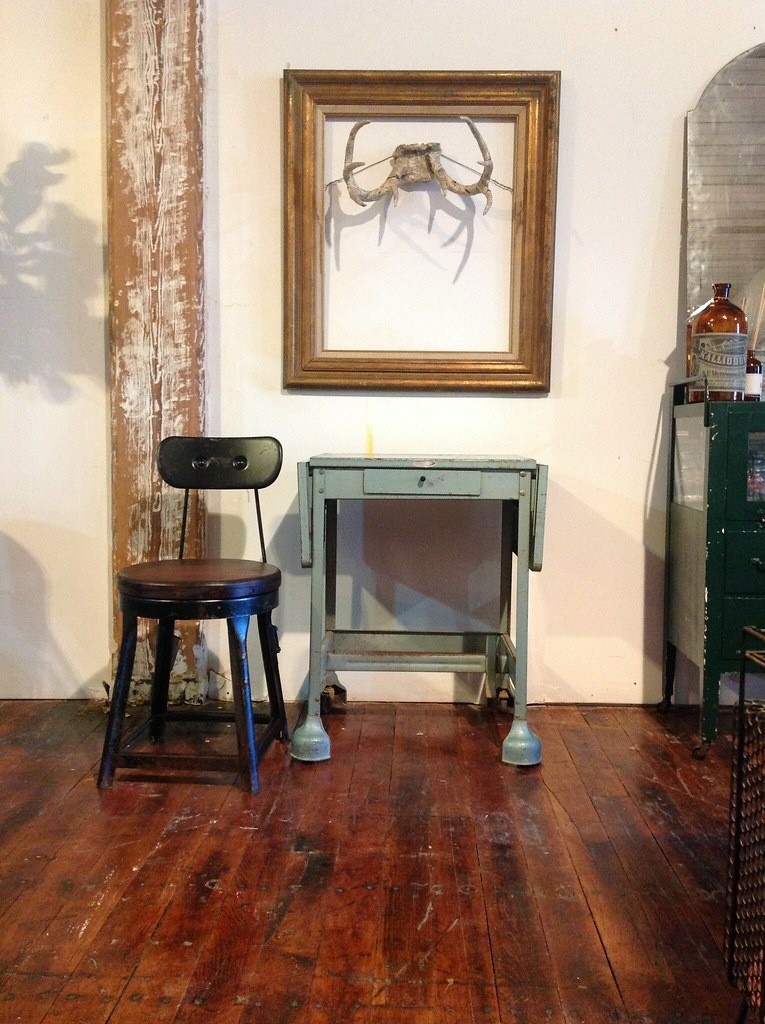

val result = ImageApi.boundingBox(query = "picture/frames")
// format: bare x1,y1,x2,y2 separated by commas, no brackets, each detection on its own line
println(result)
283,66,562,393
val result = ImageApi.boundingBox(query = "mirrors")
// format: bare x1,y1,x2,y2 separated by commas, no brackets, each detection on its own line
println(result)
685,40,764,370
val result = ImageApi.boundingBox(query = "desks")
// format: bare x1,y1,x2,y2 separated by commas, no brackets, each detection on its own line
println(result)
289,452,553,765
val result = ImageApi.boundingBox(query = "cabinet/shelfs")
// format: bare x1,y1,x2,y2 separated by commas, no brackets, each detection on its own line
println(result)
658,376,764,755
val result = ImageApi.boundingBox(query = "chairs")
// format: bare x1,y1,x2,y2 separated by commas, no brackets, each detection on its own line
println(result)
97,436,289,793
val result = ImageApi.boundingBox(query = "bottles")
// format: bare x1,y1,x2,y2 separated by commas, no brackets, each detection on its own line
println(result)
687,283,746,402
746,347,761,402
687,306,701,378
